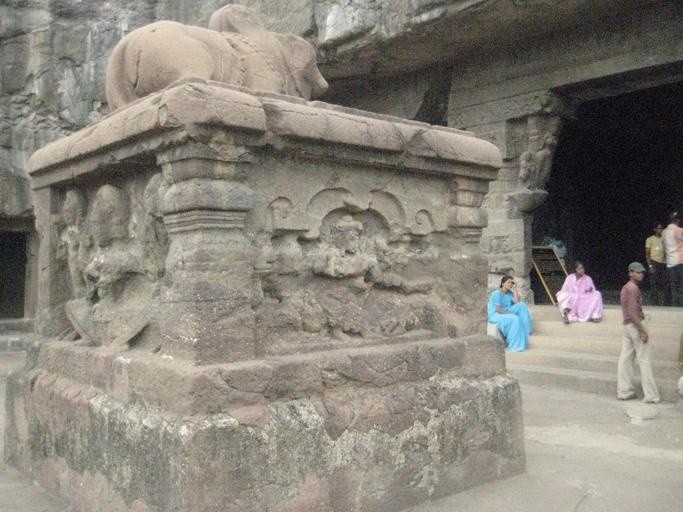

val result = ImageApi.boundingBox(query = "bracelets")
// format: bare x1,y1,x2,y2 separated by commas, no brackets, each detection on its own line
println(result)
648,264,655,268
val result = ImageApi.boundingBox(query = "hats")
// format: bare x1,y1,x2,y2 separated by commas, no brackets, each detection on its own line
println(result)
628,263,646,273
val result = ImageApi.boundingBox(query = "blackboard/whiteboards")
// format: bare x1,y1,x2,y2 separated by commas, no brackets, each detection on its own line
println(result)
531,246,567,304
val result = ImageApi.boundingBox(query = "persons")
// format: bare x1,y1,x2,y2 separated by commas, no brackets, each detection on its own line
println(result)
660,210,683,307
644,222,674,305
554,260,605,324
615,262,661,402
487,274,534,353
